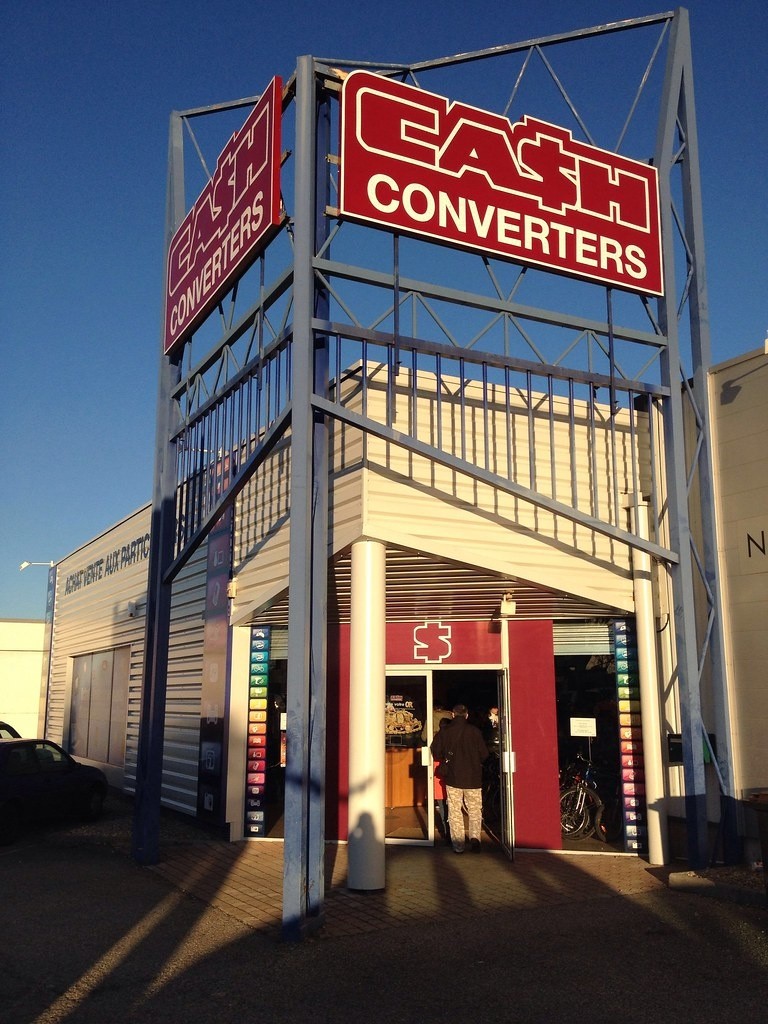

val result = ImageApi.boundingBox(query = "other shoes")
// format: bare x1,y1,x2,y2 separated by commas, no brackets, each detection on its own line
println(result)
454,850,464,855
471,838,481,853
447,840,452,847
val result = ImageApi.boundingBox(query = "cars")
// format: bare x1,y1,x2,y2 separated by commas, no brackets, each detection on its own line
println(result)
0,721,108,845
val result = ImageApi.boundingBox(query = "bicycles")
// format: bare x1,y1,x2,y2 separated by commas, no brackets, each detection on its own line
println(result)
486,737,622,844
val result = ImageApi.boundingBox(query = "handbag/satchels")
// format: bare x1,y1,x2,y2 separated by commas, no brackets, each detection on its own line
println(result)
434,752,455,778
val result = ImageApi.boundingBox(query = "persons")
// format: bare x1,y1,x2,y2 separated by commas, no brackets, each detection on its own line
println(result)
420,705,499,838
431,704,489,854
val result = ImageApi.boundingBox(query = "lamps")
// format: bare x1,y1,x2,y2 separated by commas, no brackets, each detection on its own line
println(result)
21,561,55,571
499,593,515,617
